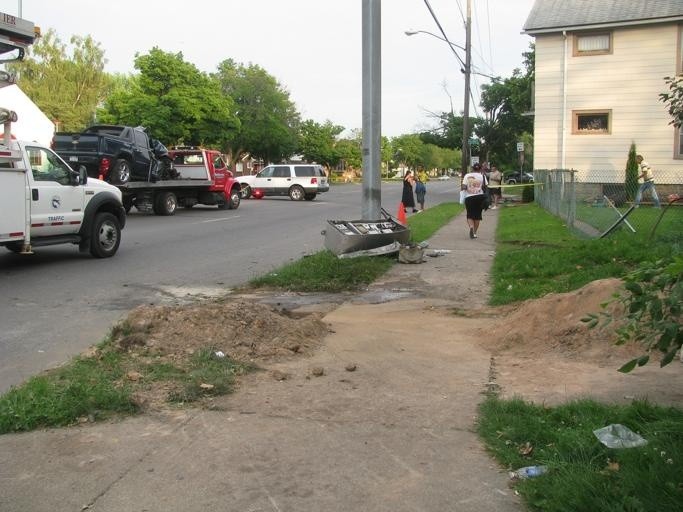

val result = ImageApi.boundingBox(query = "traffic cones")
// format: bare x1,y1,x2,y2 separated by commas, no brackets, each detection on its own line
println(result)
397,202,407,224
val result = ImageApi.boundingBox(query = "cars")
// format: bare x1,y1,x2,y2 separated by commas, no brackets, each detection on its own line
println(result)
437,175,449,180
505,173,534,184
234,164,329,201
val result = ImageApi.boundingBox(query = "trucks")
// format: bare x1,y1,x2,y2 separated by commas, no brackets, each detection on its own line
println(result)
122,145,240,216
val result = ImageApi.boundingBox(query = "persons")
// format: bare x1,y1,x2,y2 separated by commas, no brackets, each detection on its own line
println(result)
481,163,492,209
402,170,419,212
630,155,662,210
461,162,488,239
488,164,501,210
413,164,429,211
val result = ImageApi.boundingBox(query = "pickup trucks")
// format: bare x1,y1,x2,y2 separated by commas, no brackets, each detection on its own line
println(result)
1,140,127,260
48,124,161,185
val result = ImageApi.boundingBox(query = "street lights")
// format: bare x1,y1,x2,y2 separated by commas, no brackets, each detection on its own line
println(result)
405,29,471,179
